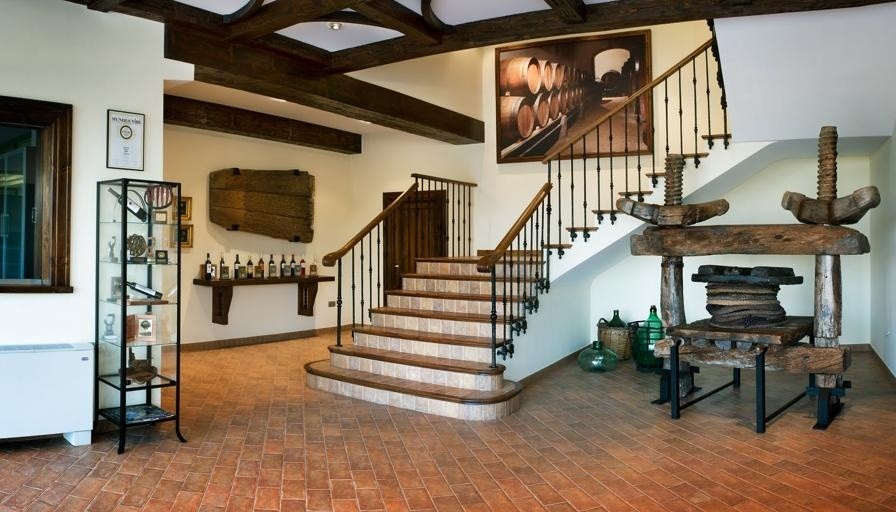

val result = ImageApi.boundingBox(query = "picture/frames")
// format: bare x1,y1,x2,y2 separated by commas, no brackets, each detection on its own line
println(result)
172,197,192,221
107,110,145,171
496,28,653,164
170,224,194,248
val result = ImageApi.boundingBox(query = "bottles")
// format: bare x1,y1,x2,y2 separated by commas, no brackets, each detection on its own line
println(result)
577,340,618,373
204,252,210,280
258,252,317,277
219,254,225,278
233,254,240,278
106,186,149,222
126,280,162,300
605,308,628,328
246,255,253,278
637,304,666,366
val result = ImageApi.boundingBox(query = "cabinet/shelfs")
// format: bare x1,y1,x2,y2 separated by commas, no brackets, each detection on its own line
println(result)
97,178,189,454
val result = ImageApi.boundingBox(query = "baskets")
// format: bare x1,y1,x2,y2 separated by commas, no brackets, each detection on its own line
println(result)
596,318,631,361
626,320,667,373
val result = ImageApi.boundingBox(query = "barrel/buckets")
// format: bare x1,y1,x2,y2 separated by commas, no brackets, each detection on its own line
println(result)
606,310,627,327
638,305,663,363
500,56,648,138
